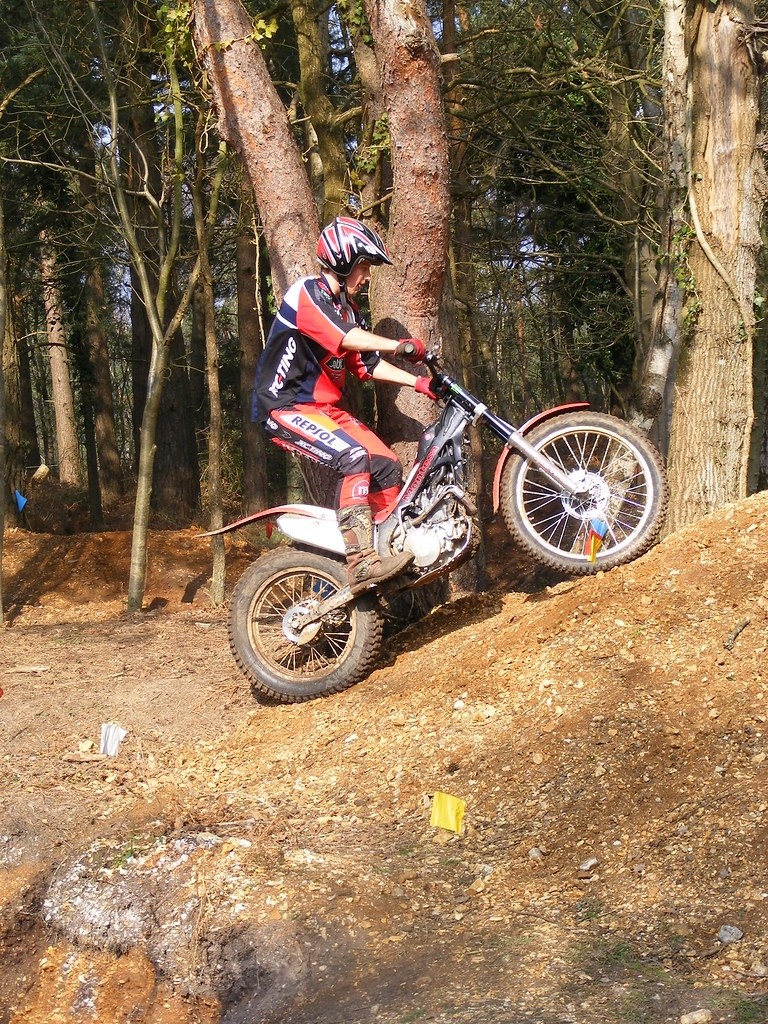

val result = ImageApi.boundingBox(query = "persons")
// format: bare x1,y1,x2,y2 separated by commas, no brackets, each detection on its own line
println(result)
250,217,451,594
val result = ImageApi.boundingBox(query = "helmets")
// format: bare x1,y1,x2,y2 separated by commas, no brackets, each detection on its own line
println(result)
317,217,393,276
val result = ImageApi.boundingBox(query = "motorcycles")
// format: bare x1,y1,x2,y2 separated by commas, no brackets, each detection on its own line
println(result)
192,340,671,703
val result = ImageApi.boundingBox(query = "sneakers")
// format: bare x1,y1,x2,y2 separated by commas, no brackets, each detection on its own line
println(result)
349,551,416,594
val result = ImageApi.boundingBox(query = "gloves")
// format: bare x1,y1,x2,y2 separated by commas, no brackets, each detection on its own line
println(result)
396,339,427,368
416,375,448,401
